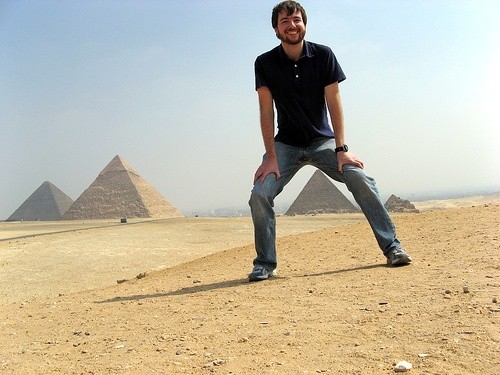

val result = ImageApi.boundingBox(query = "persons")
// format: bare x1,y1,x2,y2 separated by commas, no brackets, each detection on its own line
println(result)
248,0,412,281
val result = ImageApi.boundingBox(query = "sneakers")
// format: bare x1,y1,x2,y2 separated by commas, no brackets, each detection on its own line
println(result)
247,264,277,281
387,246,411,265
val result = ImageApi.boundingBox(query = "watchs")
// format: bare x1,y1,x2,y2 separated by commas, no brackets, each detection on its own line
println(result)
335,144,348,153
